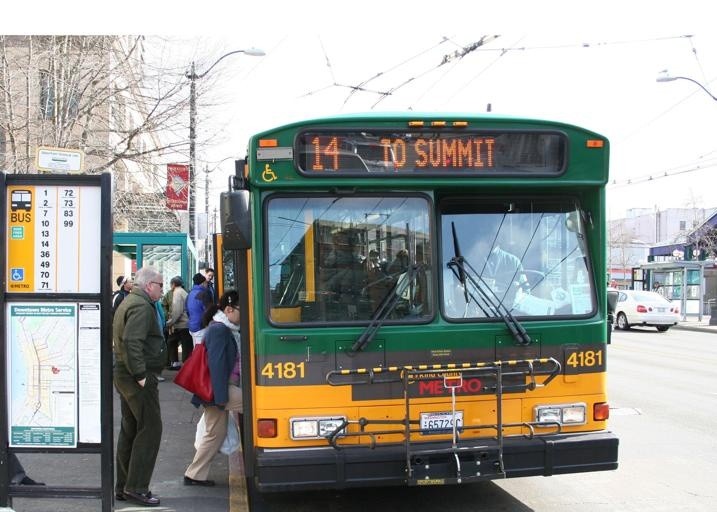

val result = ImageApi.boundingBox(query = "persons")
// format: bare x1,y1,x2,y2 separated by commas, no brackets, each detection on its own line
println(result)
312,225,531,320
608,280,618,290
653,281,664,296
110,266,243,507
7,451,47,486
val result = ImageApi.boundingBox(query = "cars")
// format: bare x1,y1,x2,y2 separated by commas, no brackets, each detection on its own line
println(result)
605,290,681,332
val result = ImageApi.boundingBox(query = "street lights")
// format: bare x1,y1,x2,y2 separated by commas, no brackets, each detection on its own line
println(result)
183,48,269,285
629,205,662,245
654,76,717,102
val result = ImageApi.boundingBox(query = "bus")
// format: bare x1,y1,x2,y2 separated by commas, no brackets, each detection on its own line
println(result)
213,112,624,494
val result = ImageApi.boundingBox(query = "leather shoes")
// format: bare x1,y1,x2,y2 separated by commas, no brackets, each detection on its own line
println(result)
184,474,215,488
116,489,161,505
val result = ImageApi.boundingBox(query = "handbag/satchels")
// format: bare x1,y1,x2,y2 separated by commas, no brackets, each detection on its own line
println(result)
173,336,213,403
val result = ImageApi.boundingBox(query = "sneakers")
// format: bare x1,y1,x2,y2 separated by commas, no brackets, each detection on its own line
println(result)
156,376,165,382
12,476,46,485
166,365,181,370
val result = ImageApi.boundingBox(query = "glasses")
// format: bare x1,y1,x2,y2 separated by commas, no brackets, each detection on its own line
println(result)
150,281,164,288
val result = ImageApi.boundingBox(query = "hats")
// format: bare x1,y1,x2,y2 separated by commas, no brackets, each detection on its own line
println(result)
192,273,206,285
369,249,379,257
116,275,129,287
